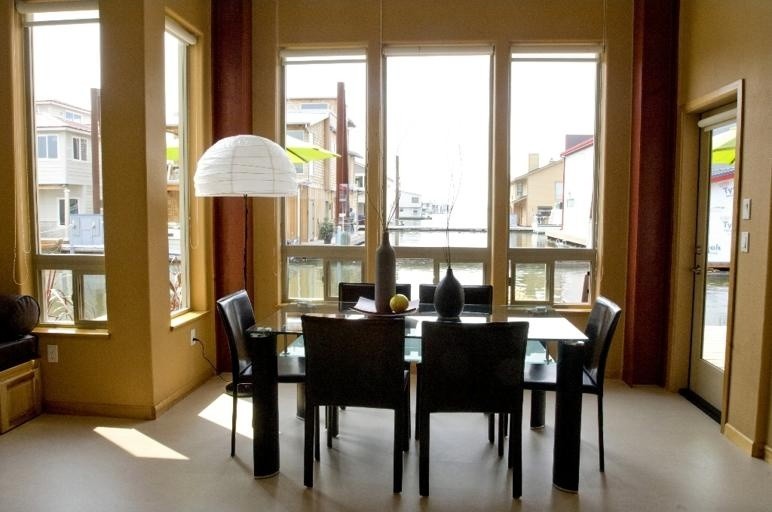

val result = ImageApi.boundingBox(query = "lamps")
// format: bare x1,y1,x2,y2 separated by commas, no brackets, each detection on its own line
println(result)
191,132,299,402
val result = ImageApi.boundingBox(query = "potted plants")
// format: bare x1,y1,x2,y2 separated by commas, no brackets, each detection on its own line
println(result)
363,165,412,313
432,198,467,322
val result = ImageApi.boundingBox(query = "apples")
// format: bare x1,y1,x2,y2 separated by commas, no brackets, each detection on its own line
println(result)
390,294,409,313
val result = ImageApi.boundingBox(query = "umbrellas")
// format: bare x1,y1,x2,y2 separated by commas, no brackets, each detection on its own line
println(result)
710,130,737,165
168,133,342,166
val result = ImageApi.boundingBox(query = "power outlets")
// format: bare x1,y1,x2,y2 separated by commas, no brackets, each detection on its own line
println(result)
191,329,196,345
48,344,58,362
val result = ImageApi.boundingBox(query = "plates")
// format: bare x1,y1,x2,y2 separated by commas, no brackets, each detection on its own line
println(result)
350,296,420,318
